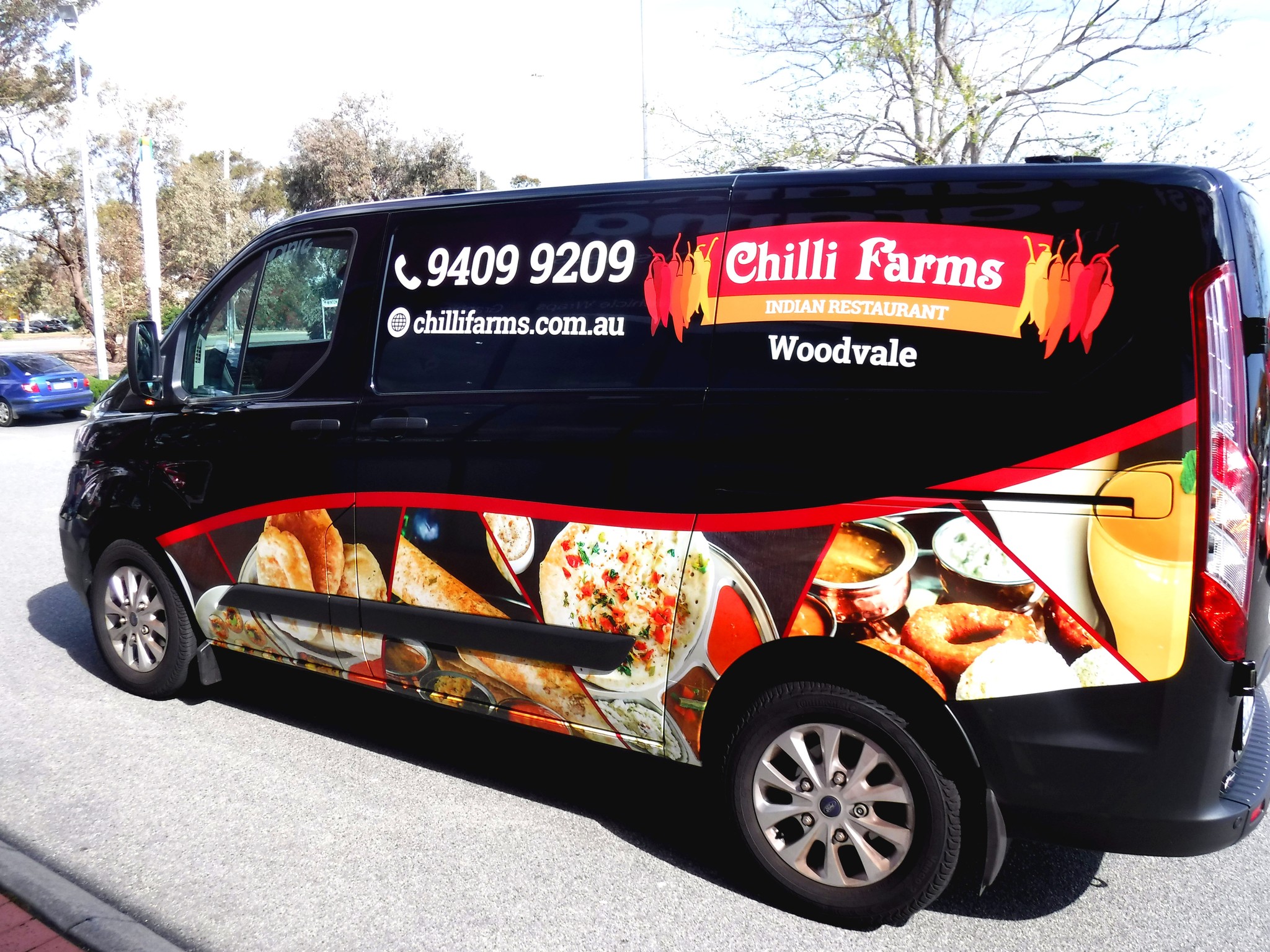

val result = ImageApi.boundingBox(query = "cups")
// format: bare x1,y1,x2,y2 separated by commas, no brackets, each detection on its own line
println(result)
1085,460,1196,680
931,518,1037,608
790,589,837,636
812,518,919,624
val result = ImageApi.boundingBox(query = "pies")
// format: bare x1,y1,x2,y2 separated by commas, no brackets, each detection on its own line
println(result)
256,508,387,658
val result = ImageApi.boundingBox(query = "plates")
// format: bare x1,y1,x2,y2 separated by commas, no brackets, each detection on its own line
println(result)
418,670,496,716
196,584,288,656
582,541,780,766
236,540,380,673
496,698,575,736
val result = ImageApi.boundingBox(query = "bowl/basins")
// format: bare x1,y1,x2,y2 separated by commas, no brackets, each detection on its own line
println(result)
382,637,432,677
483,513,535,576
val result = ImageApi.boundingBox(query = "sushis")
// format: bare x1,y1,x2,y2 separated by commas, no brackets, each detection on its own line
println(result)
208,606,283,663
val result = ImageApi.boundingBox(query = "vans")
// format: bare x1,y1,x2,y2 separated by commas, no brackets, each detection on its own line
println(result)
46,149,1270,927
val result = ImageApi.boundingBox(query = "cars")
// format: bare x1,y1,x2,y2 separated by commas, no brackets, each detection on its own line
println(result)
9,321,41,334
0,353,94,433
51,318,74,332
0,322,18,334
30,320,61,333
